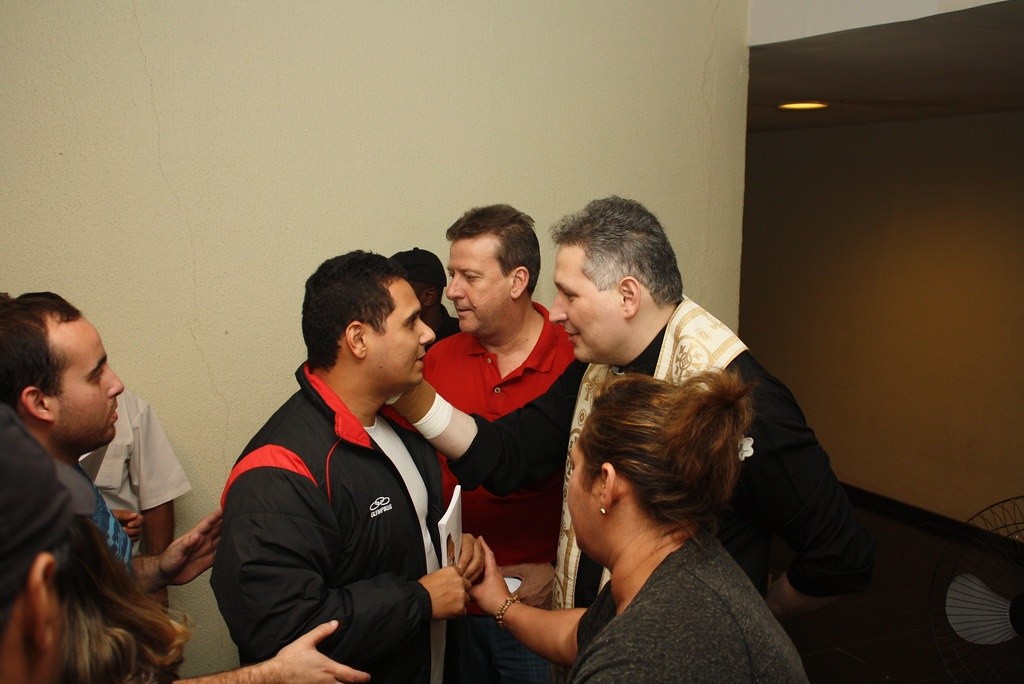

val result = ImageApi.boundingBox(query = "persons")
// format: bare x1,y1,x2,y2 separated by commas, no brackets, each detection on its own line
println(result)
447,534,457,567
389,247,460,354
1,292,192,608
384,197,873,684
469,372,809,684
0,413,189,684
210,249,485,684
380,205,576,684
0,291,370,684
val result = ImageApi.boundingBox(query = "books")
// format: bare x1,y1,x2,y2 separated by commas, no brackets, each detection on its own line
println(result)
438,485,463,568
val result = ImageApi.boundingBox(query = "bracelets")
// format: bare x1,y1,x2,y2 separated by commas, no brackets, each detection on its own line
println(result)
496,594,522,632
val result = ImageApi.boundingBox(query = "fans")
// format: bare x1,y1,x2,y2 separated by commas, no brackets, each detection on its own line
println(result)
930,497,1024,684
